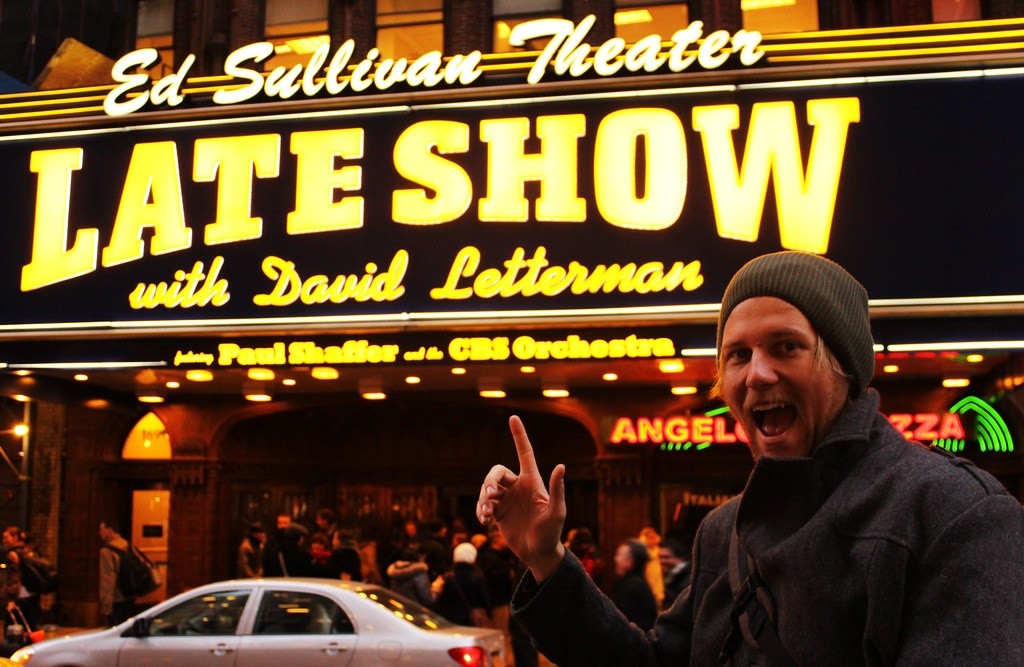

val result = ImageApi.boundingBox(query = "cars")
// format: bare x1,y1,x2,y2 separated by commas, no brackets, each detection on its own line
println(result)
7,574,510,667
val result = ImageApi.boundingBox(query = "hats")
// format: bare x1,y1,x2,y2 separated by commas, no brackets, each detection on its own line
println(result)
714,251,876,395
453,542,477,563
285,523,308,535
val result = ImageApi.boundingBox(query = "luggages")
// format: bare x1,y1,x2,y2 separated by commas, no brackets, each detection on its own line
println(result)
7,607,45,645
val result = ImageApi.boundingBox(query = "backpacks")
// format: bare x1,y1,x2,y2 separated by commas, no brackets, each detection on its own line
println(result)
106,541,163,605
13,547,60,596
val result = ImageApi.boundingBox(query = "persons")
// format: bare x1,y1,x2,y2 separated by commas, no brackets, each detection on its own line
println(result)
0,527,39,644
98,518,135,627
474,251,1024,667
240,515,692,666
